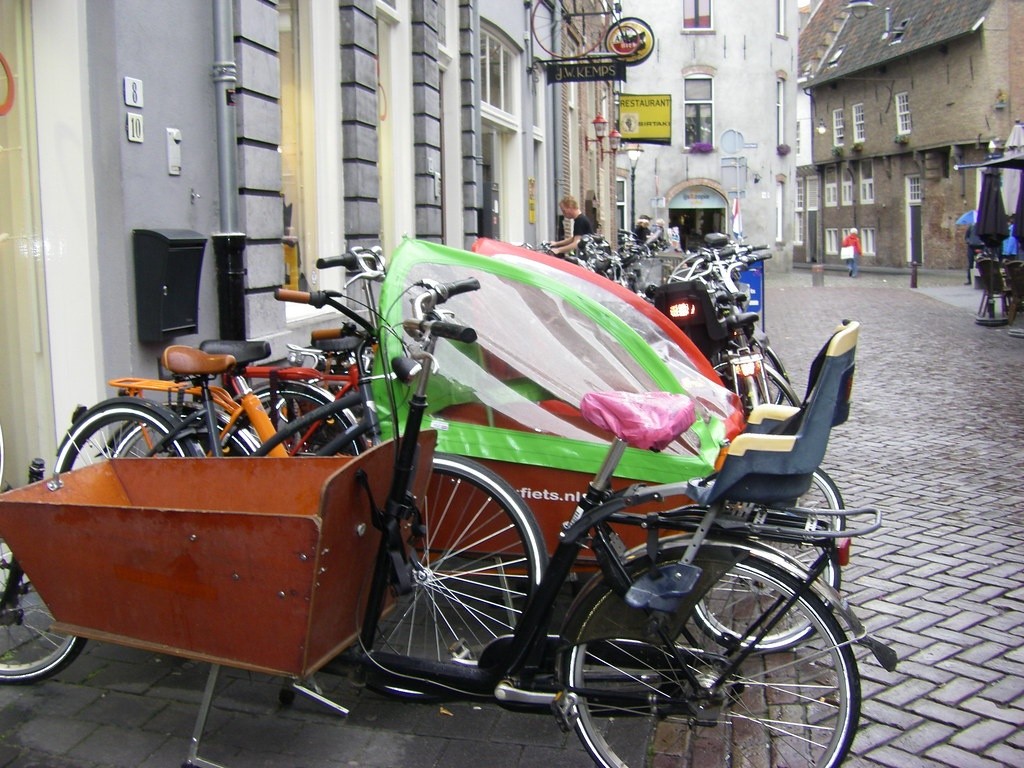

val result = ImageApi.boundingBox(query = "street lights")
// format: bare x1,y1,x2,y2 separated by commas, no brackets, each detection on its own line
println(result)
625,142,645,230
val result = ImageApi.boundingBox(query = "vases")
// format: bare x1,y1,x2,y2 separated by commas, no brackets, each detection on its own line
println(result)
994,105,1007,108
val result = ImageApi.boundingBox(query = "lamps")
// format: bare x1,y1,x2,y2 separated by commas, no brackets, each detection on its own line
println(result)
601,126,623,162
586,112,609,162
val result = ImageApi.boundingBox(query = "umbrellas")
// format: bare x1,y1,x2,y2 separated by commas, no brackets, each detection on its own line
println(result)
955,210,979,225
975,153,1009,327
1006,169,1024,338
1006,119,1024,155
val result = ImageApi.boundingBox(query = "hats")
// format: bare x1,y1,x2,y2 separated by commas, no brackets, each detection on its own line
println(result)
851,228,857,234
639,215,652,222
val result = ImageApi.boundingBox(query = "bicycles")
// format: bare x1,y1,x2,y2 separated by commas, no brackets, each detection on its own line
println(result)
0,228,900,768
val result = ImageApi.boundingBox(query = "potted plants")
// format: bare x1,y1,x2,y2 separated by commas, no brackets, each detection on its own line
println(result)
851,143,862,151
894,136,909,143
831,147,843,156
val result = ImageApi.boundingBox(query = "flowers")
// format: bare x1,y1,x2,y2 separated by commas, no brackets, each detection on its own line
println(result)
776,143,790,155
992,87,1009,104
689,142,713,154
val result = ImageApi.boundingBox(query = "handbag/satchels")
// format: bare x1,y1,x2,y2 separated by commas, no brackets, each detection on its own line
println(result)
840,246,854,260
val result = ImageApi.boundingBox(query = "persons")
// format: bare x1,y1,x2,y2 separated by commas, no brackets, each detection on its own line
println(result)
964,223,985,285
840,228,861,278
547,198,595,256
1002,214,1020,261
635,214,650,244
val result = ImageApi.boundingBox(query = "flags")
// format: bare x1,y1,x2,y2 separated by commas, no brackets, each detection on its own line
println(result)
732,198,743,241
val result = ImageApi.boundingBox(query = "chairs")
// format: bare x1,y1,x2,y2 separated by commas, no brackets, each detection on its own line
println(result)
975,256,1024,325
701,320,859,503
